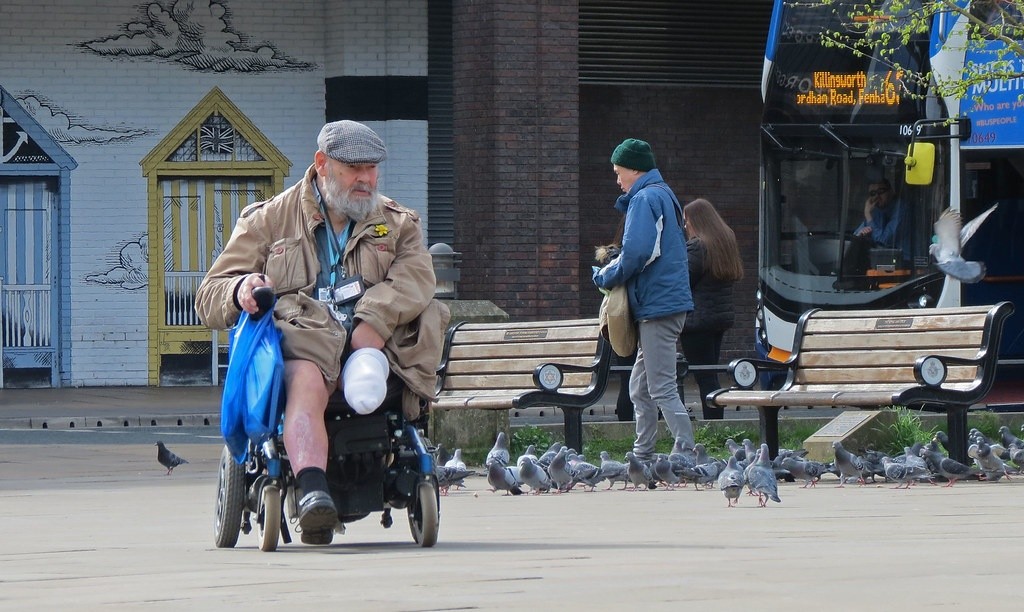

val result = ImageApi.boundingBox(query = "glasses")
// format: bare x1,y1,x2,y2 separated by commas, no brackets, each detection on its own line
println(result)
870,187,890,196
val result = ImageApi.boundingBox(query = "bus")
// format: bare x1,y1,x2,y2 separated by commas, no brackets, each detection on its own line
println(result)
752,1,1024,396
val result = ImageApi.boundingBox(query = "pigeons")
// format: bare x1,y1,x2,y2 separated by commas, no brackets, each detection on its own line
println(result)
435,426,1024,497
748,444,781,509
154,440,189,476
718,457,745,508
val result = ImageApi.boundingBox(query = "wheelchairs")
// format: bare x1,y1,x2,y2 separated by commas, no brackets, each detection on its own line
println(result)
212,285,441,552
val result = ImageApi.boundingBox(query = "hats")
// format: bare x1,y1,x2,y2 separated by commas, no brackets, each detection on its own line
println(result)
610,138,655,172
316,119,387,164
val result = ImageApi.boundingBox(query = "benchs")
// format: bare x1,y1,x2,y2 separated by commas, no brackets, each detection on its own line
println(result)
426,313,610,462
705,301,1019,471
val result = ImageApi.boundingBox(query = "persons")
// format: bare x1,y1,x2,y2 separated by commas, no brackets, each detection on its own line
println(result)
192,119,451,549
593,214,665,421
678,198,746,421
592,139,699,481
850,174,912,258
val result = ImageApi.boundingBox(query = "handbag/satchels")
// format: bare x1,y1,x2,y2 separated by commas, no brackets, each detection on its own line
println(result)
607,285,638,356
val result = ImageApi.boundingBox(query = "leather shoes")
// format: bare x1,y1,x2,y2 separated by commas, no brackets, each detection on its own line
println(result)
298,491,337,545
